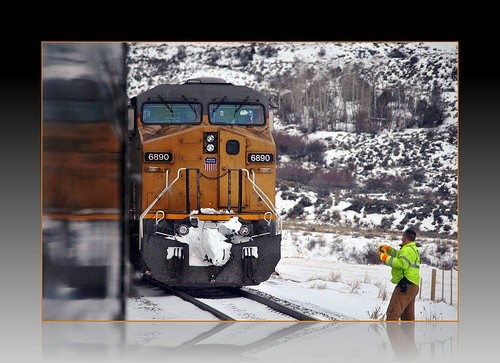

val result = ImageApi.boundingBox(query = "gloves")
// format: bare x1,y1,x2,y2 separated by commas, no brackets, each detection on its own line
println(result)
377,244,390,265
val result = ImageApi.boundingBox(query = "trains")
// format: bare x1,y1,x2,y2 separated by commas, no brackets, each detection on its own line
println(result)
126,77,283,293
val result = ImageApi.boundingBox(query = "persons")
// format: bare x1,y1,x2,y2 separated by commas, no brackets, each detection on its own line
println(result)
377,229,421,363
